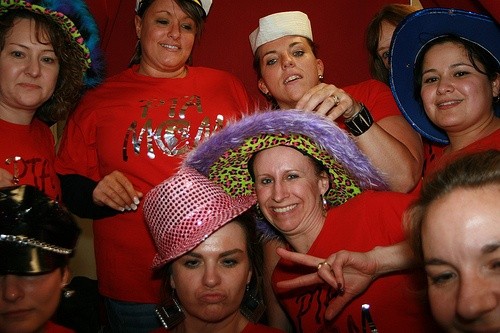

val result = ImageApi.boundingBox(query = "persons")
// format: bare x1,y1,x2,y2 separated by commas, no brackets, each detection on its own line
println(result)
362,3,427,89
58,0,254,333
246,145,437,333
274,13,499,320
143,166,292,333
246,10,423,195
1,0,91,205
0,183,82,333
404,149,500,332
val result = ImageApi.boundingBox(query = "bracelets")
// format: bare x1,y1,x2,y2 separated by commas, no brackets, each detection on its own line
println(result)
343,101,374,136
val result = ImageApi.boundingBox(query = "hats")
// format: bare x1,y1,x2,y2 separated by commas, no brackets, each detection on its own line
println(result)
181,108,390,245
387,6,500,143
248,11,314,54
144,168,258,270
0,1,107,87
134,0,213,18
0,184,85,275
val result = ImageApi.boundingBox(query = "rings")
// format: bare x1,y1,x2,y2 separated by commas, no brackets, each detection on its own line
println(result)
331,94,340,105
316,260,332,271
